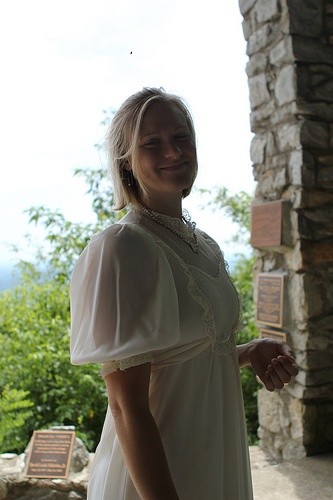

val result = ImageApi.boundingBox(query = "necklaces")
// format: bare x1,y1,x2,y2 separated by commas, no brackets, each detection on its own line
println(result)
139,202,200,254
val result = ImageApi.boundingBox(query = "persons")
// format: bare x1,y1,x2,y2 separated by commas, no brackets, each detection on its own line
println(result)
69,86,301,500
